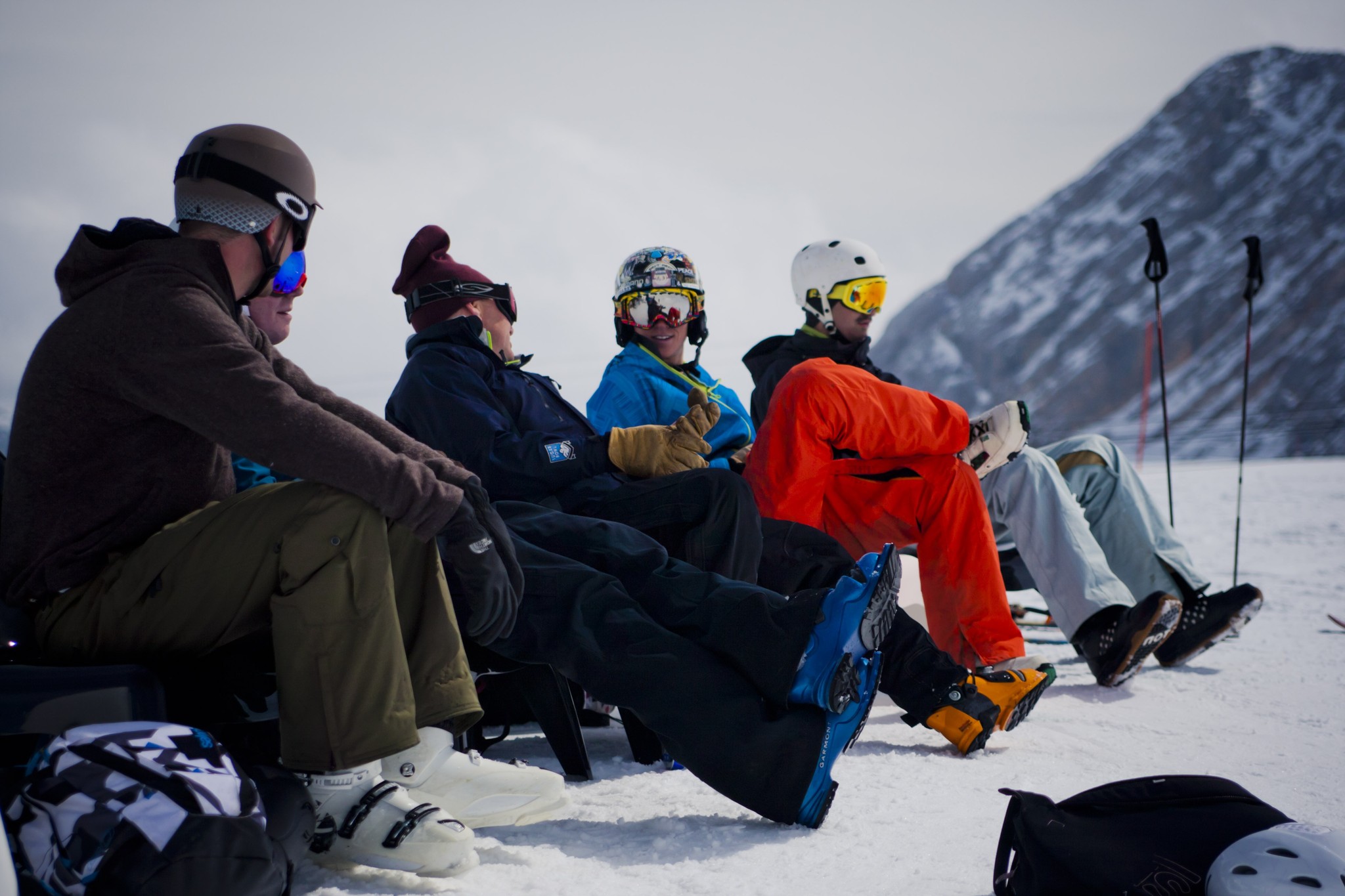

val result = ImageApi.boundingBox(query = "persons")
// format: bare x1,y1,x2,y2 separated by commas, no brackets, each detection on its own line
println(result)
1,122,566,872
583,243,1042,670
232,250,904,829
389,226,1058,753
739,240,1265,688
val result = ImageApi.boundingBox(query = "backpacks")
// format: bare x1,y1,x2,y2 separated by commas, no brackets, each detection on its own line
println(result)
993,776,1297,895
0,721,294,896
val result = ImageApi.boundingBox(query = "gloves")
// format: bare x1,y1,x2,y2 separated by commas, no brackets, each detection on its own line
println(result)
459,476,525,608
436,494,519,647
608,425,712,479
659,387,721,439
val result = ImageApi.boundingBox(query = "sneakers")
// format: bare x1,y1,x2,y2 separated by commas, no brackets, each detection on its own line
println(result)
954,400,1031,480
294,726,566,884
785,542,1264,831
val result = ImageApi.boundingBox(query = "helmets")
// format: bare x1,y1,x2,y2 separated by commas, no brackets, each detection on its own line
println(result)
173,124,324,235
612,246,704,307
790,239,886,307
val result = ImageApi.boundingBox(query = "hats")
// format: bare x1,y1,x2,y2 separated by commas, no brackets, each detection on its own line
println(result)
392,226,494,333
